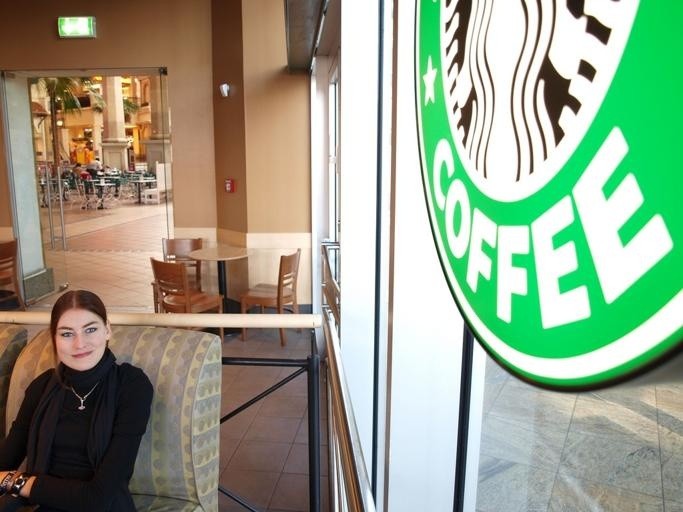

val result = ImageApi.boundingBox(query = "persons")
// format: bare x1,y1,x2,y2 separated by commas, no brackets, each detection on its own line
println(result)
81,169,93,188
71,162,83,180
0,290,155,512
87,157,104,179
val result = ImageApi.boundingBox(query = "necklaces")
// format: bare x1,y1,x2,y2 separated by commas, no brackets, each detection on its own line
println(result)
69,379,102,411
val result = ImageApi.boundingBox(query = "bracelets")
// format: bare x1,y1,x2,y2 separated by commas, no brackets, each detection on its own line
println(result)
11,473,31,498
1,472,16,490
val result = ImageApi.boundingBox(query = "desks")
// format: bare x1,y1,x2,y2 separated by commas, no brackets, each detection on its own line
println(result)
39,171,154,210
185,244,254,335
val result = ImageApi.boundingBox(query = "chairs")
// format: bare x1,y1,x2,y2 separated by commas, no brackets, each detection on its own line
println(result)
240,249,302,348
151,234,226,345
0,237,26,311
143,161,160,205
1,321,223,510
